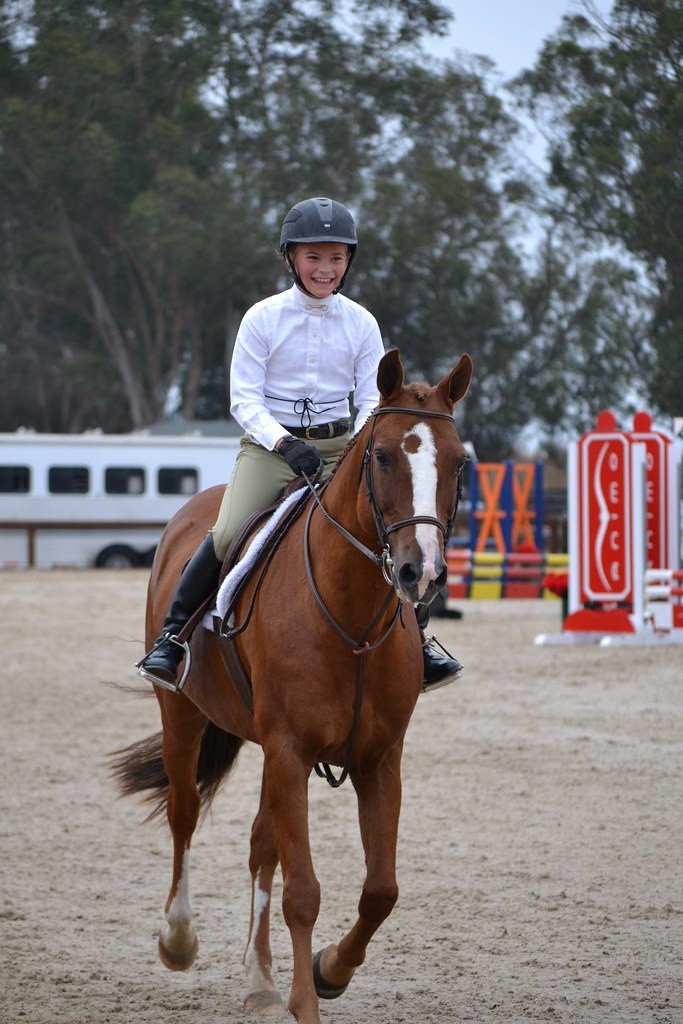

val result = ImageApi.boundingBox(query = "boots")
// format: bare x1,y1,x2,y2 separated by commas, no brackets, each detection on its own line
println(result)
142,531,233,681
415,579,462,684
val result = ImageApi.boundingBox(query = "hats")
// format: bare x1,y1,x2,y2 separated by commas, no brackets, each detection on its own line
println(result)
278,197,359,254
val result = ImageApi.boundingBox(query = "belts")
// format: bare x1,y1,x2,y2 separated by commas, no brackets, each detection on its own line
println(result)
281,418,350,440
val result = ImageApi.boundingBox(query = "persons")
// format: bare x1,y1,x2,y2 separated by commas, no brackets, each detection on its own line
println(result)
144,197,461,684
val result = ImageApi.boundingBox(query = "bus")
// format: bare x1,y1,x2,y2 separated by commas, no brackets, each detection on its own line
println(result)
1,423,244,573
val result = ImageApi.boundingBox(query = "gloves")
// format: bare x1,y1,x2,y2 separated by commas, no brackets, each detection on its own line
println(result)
277,435,321,477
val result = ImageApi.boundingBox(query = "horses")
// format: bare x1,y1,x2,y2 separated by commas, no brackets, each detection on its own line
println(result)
99,348,475,1022
